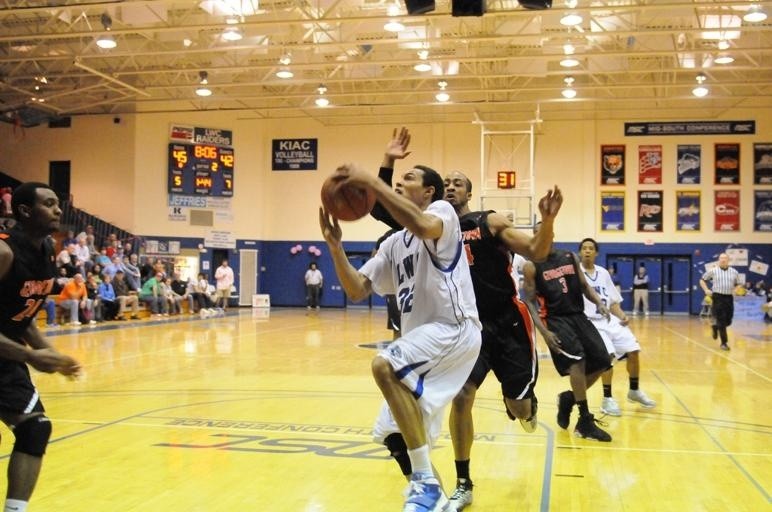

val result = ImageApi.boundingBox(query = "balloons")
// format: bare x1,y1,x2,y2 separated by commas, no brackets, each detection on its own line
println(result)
315,248,321,257
296,245,302,251
291,246,297,255
308,245,317,253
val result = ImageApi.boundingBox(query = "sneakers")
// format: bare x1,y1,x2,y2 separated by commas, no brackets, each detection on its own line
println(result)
306,306,311,312
633,311,638,315
645,312,650,315
520,416,537,433
316,306,320,310
627,389,656,407
403,472,473,512
712,326,718,339
720,344,729,350
557,391,576,429
35,307,229,325
601,397,622,416
575,413,611,441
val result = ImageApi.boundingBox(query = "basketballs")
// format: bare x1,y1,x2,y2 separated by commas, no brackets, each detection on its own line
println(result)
321,172,371,222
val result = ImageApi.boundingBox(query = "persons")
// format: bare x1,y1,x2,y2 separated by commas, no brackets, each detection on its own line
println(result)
301,263,326,309
367,125,565,512
371,220,652,444
0,178,85,512
317,163,485,512
698,250,744,352
0,182,239,326
745,280,772,302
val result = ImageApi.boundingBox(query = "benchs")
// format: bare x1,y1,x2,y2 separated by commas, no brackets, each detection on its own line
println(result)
33,299,199,329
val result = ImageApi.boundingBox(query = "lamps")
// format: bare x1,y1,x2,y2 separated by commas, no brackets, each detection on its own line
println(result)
95,14,117,49
314,84,331,106
195,70,211,98
690,74,711,97
435,79,450,102
743,1,768,24
559,76,579,100
